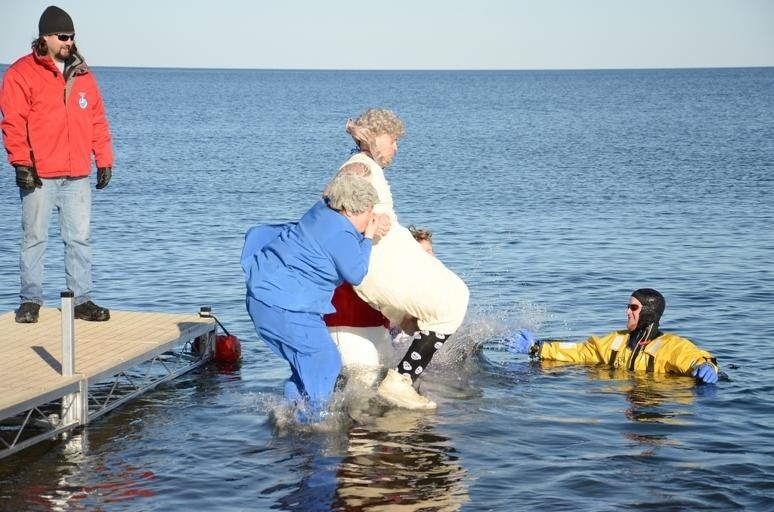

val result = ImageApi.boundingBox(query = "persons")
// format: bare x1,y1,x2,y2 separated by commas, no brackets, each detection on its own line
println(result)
504,287,718,386
324,224,436,342
336,110,471,410
0,4,114,323
238,175,391,424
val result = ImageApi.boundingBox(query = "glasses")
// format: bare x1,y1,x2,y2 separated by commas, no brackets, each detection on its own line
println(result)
627,302,639,311
57,34,75,41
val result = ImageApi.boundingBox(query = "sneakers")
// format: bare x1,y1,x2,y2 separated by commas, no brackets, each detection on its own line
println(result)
16,303,40,322
74,301,110,321
378,369,438,413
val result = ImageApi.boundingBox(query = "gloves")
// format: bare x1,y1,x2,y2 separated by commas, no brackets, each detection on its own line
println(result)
16,165,42,190
96,167,111,189
505,328,535,353
690,361,718,386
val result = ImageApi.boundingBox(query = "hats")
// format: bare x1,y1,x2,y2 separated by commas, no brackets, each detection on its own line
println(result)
39,6,74,34
